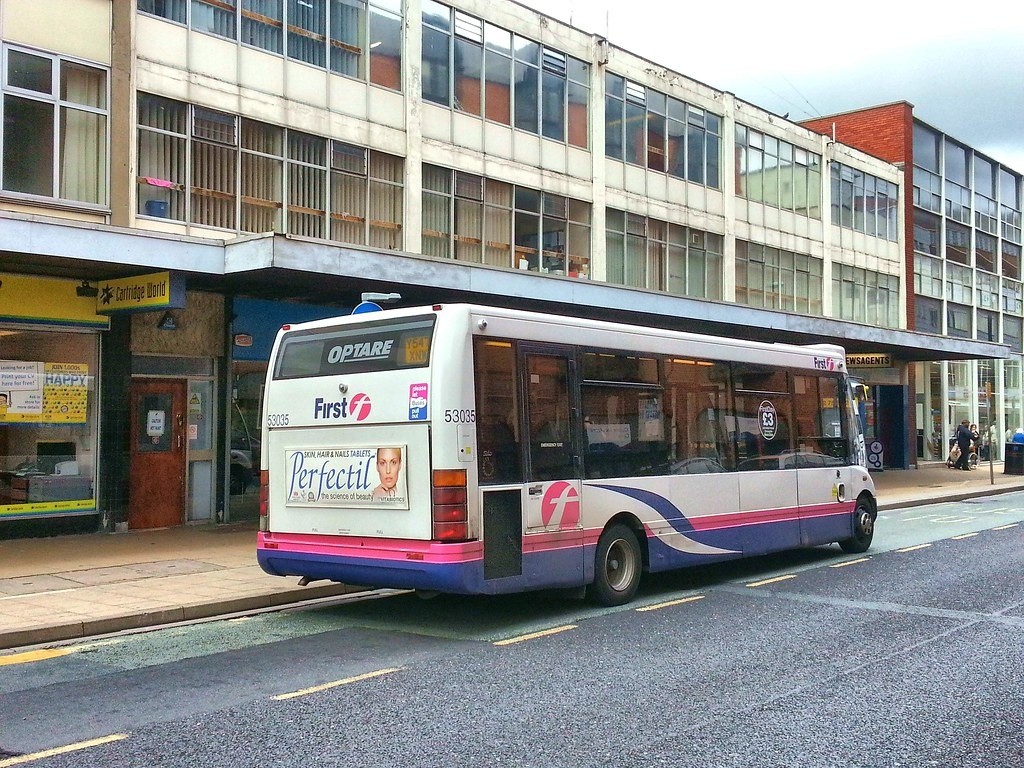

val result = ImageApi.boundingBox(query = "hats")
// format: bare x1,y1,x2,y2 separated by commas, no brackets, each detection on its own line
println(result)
962,419,970,423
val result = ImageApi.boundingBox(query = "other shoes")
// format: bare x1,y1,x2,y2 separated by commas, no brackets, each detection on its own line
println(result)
963,468,971,471
954,465,961,470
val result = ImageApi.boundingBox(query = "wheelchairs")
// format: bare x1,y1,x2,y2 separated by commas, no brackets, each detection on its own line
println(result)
947,446,980,470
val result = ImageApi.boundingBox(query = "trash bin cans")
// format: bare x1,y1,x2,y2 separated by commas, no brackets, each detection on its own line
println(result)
1003,441,1024,475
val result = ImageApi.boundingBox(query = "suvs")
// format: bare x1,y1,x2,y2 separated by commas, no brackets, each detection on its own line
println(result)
737,448,847,470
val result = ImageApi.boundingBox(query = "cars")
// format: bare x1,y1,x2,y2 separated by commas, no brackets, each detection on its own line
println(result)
587,441,635,454
231,450,252,495
640,456,727,476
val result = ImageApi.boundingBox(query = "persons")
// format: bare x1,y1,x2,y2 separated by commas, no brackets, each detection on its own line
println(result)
983,419,1012,459
374,448,401,505
954,420,979,470
1012,428,1024,443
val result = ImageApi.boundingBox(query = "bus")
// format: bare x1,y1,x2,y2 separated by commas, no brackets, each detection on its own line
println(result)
256,302,878,607
